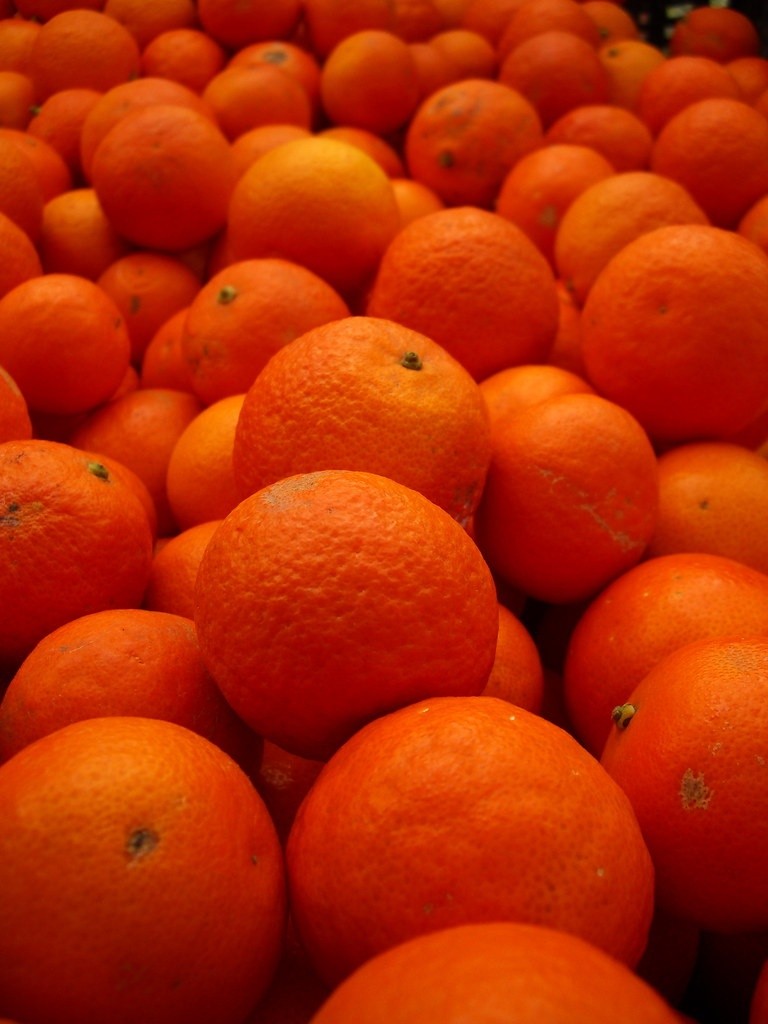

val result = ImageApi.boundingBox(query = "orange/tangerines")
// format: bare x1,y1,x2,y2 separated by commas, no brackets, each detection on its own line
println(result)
0,0,767,1024
